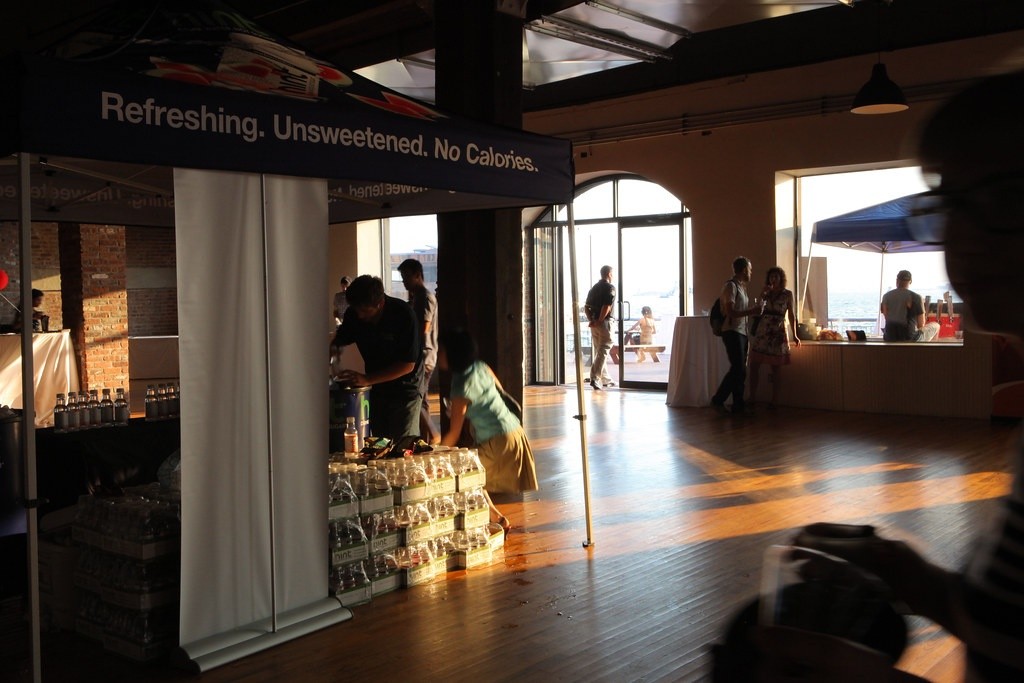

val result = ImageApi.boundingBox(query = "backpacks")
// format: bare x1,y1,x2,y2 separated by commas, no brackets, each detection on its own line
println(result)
709,280,739,336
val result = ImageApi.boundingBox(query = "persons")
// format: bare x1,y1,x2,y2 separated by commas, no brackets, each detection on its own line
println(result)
397,259,441,446
883,270,940,343
334,276,352,329
628,306,660,362
437,327,538,541
584,265,618,390
12,289,43,333
745,268,798,409
709,256,763,417
706,70,1024,683
329,275,425,448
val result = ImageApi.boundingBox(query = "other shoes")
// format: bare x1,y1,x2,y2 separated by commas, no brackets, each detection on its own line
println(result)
428,435,442,446
731,406,759,418
604,382,614,387
590,380,602,391
709,401,733,418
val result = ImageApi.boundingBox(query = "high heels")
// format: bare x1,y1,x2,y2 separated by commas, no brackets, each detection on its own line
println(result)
499,516,512,541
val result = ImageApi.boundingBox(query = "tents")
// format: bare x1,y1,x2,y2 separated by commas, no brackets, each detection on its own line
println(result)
800,190,943,335
0,0,591,683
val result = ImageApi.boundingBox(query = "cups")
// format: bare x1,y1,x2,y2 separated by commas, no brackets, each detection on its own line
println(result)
701,306,711,316
954,331,964,339
755,298,763,307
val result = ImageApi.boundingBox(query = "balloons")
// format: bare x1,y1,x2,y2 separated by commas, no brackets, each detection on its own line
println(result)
0,269,9,291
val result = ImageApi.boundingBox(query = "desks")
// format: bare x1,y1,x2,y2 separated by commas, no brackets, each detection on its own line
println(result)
0,328,80,424
128,335,179,379
36,416,180,522
666,316,750,405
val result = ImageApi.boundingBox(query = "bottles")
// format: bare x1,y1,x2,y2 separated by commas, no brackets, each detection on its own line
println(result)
325,445,490,592
73,491,180,645
67,392,79,428
114,388,128,425
76,391,89,427
101,389,114,427
145,381,180,422
54,394,68,431
88,389,101,427
344,417,358,458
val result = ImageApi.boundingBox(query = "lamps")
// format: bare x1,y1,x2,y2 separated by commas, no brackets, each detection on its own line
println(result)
851,45,910,115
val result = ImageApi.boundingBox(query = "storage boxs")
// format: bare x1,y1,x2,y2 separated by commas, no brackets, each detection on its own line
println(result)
69,468,505,662
